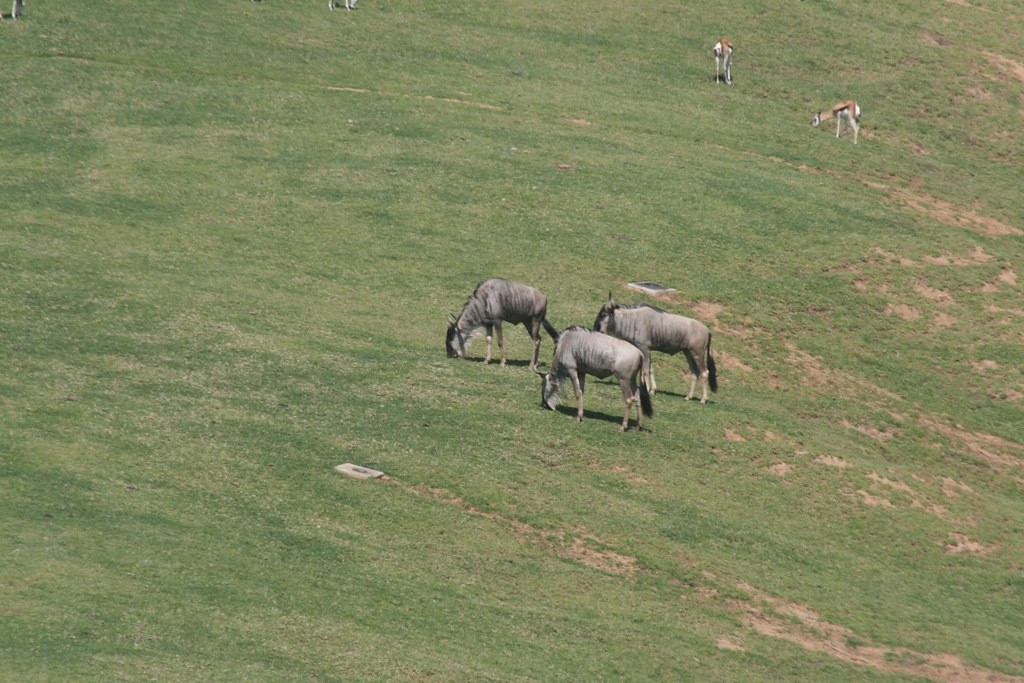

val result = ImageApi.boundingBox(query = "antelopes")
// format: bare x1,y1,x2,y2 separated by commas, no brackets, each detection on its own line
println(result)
809,99,863,146
712,37,735,86
